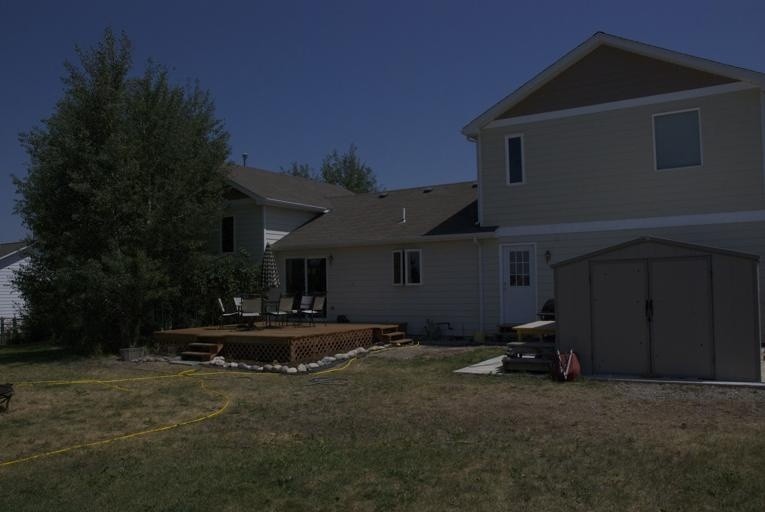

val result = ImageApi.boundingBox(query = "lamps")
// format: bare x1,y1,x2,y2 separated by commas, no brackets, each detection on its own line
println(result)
328,253,333,267
544,251,552,265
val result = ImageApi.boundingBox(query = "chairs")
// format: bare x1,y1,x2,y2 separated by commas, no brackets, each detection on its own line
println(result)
216,290,326,330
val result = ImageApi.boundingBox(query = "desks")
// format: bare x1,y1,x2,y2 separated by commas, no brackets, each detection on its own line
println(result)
511,319,555,343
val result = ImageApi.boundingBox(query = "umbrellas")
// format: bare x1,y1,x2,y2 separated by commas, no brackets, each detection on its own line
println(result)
259,241,281,290
